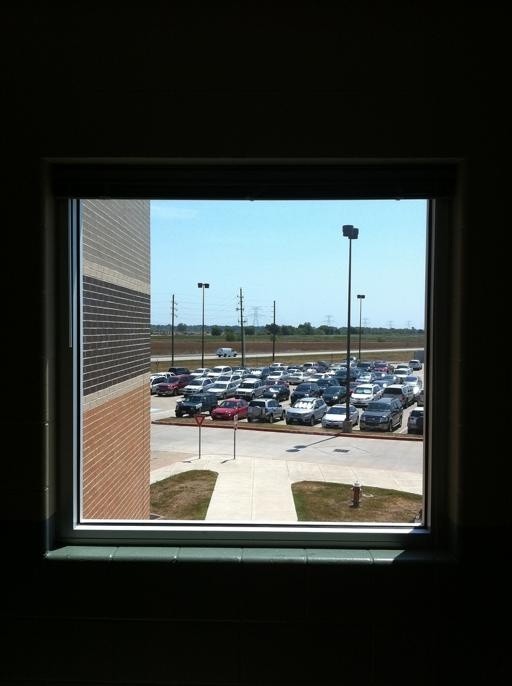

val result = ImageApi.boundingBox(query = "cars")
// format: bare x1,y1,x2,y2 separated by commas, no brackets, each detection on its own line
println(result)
150,356,423,436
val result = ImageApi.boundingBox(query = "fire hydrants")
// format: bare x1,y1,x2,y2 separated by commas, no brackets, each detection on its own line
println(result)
350,480,363,508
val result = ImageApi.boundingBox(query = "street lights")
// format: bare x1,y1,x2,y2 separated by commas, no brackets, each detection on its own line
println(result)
357,294,365,358
343,224,359,433
198,282,210,367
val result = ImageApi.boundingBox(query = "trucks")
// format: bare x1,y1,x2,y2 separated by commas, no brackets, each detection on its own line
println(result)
216,348,238,357
